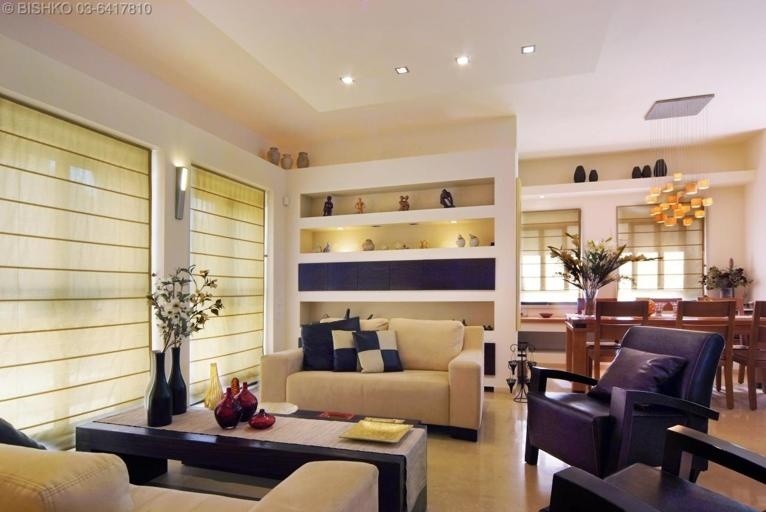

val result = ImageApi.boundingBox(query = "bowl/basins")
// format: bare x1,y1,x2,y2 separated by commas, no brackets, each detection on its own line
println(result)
540,312,554,318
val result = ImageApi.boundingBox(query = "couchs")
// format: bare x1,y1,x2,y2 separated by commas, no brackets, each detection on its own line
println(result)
0,413,380,512
523,325,724,484
260,316,483,444
533,424,765,511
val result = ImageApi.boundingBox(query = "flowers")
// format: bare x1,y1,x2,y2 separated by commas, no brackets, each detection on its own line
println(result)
701,263,750,292
158,265,219,348
546,232,662,298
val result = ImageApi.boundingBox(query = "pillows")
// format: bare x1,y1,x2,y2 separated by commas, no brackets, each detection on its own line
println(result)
353,330,399,371
300,316,356,370
331,328,360,369
1,418,46,448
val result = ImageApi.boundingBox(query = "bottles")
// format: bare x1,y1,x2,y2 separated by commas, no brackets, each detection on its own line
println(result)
203,361,275,431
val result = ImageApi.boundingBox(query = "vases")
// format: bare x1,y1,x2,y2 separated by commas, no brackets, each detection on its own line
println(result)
168,345,190,411
214,386,241,430
265,146,314,169
204,362,224,410
146,348,178,427
232,382,258,423
584,291,598,318
572,158,667,182
719,285,735,297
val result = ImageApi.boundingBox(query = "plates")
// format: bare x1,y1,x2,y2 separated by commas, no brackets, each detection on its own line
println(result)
340,417,414,444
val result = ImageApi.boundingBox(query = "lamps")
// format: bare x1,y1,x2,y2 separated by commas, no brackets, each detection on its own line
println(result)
644,92,716,227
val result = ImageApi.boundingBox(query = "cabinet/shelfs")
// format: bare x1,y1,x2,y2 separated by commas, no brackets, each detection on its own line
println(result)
299,178,498,251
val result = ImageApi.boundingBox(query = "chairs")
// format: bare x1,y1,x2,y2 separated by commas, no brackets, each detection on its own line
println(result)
564,300,766,413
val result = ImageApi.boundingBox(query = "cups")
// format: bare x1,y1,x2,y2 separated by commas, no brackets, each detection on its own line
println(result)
653,299,678,319
